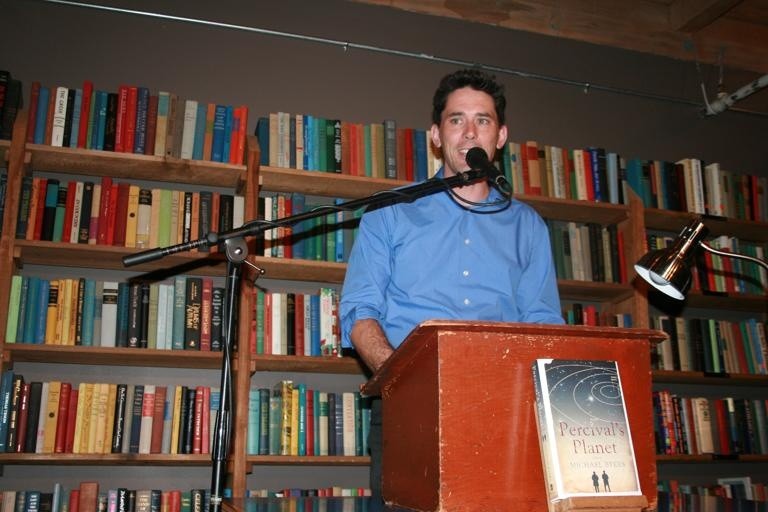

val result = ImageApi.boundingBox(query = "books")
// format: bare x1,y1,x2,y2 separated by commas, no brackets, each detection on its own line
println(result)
2,482,232,512
652,390,766,456
256,111,444,183
5,275,226,351
657,477,767,512
1,372,221,454
561,303,632,328
542,217,629,284
15,176,245,265
245,488,373,512
246,380,373,456
2,69,246,182
649,315,766,374
494,141,629,204
648,236,766,294
630,158,766,221
252,288,343,358
256,192,369,263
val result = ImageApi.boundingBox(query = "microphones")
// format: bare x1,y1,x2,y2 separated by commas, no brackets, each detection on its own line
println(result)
465,147,513,198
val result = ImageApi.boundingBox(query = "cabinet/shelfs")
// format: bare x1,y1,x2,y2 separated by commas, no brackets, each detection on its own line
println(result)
0,107,768,512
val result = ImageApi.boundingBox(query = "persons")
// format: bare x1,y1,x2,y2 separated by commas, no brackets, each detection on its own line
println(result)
339,69,564,392
